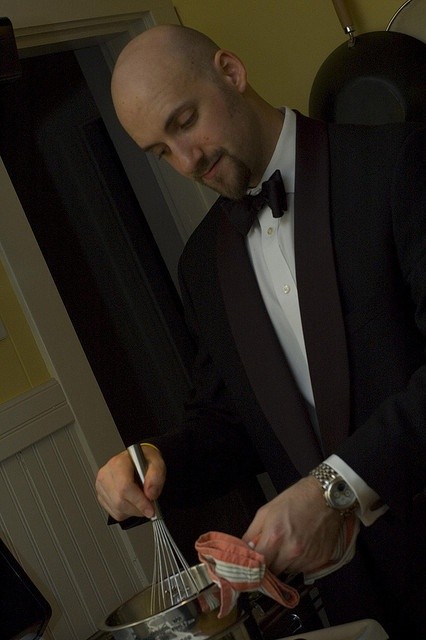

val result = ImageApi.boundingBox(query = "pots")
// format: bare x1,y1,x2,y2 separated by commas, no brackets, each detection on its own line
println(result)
308,0,426,125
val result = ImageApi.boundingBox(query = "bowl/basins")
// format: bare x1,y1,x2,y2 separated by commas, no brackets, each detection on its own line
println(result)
99,559,237,640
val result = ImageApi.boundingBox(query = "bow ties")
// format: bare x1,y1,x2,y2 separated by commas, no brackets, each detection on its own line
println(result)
217,170,289,237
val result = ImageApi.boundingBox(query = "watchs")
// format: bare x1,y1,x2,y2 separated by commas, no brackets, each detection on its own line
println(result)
311,461,359,519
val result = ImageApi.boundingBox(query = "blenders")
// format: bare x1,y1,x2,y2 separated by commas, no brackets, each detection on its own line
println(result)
126,444,206,614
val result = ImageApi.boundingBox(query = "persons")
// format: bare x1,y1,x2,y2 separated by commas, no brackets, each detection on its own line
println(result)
95,24,424,637
